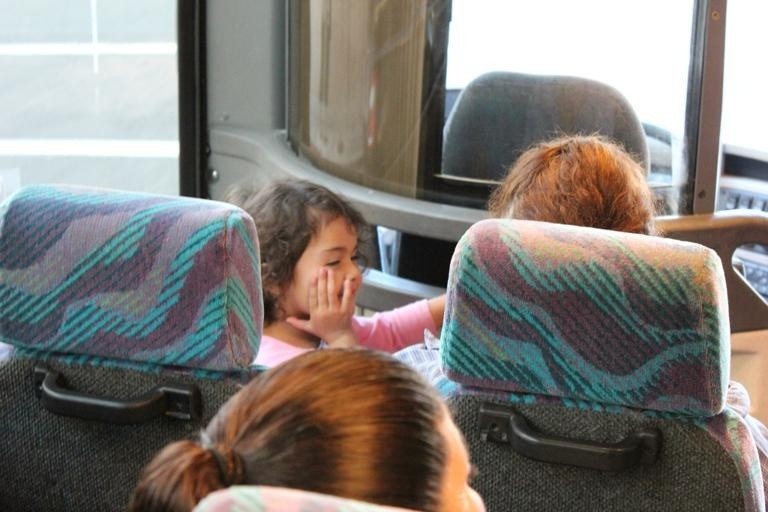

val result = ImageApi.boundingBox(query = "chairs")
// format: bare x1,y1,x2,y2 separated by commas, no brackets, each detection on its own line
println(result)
1,183,765,512
442,72,650,188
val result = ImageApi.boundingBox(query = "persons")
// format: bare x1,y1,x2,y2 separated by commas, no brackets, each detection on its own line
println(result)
419,131,768,508
130,344,486,512
224,174,448,371
